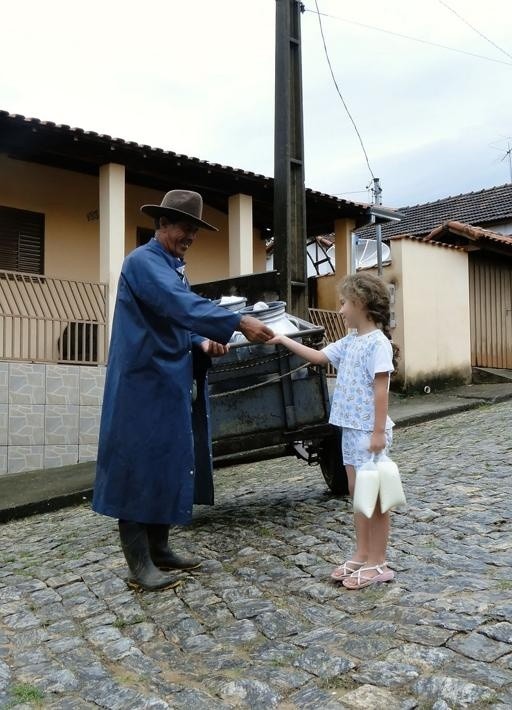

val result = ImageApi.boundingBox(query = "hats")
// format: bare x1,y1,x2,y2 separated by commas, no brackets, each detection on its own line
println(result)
137,187,221,235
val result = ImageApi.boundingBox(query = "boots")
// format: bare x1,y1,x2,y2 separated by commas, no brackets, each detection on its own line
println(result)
117,517,183,593
147,523,203,571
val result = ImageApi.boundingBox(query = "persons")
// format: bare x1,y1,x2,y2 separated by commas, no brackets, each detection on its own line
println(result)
92,190,273,591
266,273,395,591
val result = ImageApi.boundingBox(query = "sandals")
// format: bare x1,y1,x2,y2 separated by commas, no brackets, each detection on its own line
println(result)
341,561,397,590
330,558,367,581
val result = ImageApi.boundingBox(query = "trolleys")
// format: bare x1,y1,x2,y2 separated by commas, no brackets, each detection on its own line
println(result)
178,272,350,523
